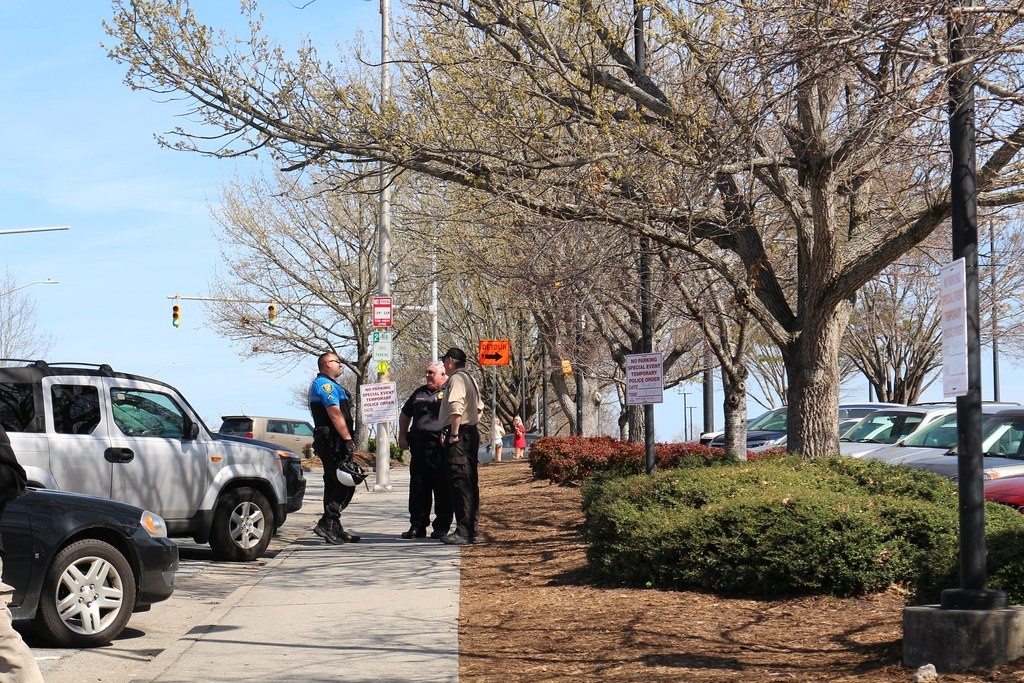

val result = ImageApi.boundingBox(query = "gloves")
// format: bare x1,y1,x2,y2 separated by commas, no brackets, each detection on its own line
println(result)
344,440,357,460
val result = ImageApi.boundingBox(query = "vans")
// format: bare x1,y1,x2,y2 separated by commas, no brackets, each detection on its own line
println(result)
218,416,314,460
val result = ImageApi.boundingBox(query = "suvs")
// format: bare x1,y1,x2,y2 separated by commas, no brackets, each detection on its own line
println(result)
701,401,1024,530
0,359,306,562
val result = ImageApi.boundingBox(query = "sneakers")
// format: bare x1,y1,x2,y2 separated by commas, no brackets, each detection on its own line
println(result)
332,519,360,543
314,514,343,545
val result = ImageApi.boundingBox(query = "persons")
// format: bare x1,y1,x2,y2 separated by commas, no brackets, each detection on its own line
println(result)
437,348,485,544
398,361,453,538
513,416,526,458
308,353,361,545
489,418,505,461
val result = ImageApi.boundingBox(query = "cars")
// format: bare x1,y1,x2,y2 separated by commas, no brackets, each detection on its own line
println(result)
478,434,544,463
0,486,179,648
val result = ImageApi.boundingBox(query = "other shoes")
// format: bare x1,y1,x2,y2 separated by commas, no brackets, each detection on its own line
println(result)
402,526,426,538
431,530,447,538
440,532,470,544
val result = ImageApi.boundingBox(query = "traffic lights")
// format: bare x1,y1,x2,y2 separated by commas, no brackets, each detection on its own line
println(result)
268,305,276,325
173,304,181,327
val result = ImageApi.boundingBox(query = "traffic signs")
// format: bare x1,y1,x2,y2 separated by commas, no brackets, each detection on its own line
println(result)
479,341,510,365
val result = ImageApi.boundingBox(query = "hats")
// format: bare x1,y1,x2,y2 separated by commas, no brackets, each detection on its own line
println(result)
438,348,467,362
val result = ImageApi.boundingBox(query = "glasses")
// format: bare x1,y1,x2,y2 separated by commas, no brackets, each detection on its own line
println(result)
425,371,437,374
323,360,340,363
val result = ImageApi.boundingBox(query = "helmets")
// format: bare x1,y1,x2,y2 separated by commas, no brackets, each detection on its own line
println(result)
336,461,369,486
